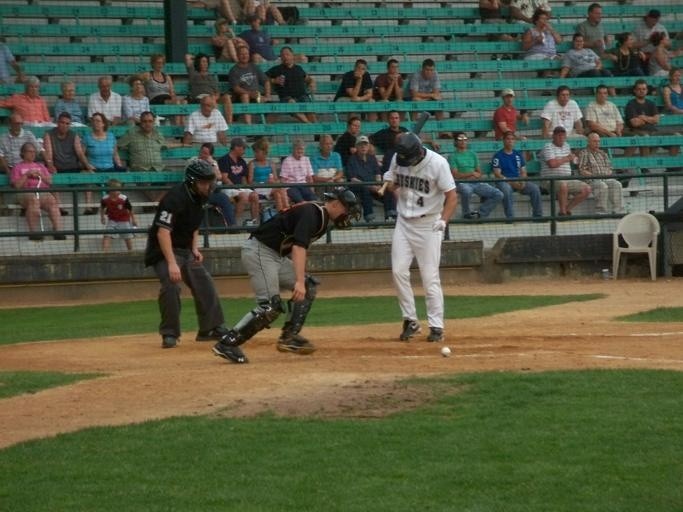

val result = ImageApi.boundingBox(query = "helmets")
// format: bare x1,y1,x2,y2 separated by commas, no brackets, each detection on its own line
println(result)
395,132,422,166
186,158,215,209
323,188,362,230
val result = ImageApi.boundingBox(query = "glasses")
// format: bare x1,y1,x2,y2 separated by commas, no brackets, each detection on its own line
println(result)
458,137,468,141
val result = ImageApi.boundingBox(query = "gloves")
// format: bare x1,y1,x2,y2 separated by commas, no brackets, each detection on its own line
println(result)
432,220,446,232
383,170,394,182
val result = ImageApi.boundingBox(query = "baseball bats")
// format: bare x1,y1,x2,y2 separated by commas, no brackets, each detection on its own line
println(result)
377,110,429,195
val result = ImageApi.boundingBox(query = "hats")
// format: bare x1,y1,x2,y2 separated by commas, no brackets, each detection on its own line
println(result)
231,138,249,148
501,88,515,97
356,136,369,144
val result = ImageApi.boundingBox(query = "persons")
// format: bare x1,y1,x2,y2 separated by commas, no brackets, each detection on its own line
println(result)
382,132,458,341
99,178,140,252
144,160,231,348
1,2,452,240
211,186,363,364
448,0,682,220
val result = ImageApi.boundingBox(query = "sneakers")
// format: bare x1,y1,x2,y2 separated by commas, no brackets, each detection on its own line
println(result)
162,336,176,347
428,328,444,342
465,211,480,218
385,217,397,222
400,319,421,341
368,219,377,229
29,236,65,240
59,208,95,215
196,327,229,342
278,336,314,354
213,341,247,364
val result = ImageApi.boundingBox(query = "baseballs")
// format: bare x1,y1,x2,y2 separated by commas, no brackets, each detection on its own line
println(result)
442,346,451,356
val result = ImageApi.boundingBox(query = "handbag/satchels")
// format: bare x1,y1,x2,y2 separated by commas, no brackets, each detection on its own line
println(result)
261,207,278,224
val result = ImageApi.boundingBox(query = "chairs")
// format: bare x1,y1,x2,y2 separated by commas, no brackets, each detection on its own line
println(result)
613,212,661,279
